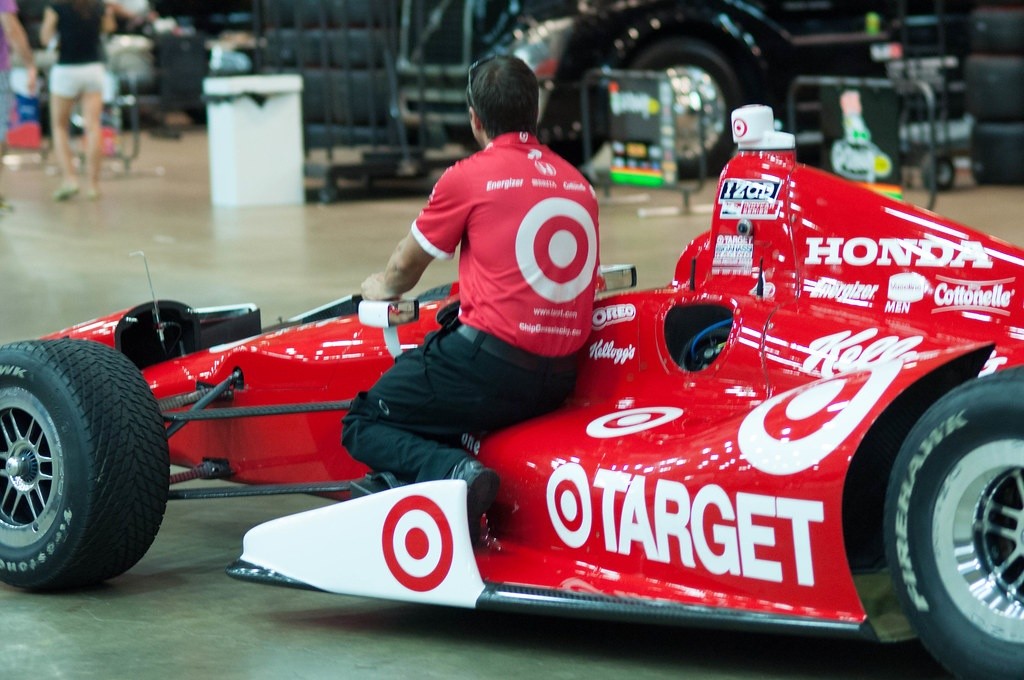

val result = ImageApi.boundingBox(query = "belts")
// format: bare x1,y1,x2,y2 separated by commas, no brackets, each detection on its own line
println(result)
457,317,577,373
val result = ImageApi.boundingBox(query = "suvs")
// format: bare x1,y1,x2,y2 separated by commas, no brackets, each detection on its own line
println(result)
393,1,972,181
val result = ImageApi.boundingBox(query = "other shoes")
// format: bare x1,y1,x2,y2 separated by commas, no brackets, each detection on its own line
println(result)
52,182,80,202
88,190,100,200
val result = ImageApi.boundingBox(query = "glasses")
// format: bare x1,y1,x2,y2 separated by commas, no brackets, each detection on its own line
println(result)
468,52,510,106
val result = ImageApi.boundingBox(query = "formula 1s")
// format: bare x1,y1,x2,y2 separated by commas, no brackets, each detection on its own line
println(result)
0,103,1024,680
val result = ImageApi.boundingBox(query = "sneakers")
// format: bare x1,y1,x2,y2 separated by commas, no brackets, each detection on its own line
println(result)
448,454,501,552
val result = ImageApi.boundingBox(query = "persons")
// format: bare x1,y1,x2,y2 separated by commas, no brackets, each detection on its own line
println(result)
0,0,167,210
339,55,602,543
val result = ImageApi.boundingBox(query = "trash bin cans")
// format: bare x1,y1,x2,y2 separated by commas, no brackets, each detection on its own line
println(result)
202,74,306,211
